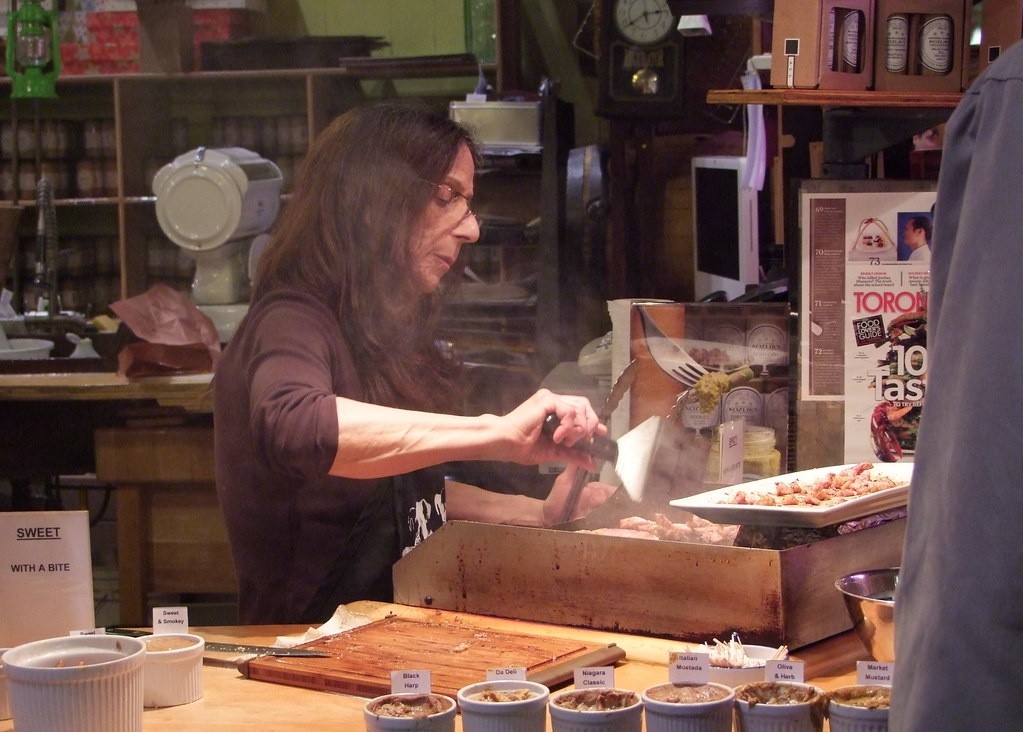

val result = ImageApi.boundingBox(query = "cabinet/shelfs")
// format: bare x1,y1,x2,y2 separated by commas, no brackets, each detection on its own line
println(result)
0,63,518,342
0,373,238,626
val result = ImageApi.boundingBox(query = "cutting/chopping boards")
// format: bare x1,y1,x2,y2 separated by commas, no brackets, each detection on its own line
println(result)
237,616,626,713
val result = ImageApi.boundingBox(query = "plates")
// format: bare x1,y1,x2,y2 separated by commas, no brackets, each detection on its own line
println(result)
669,461,914,529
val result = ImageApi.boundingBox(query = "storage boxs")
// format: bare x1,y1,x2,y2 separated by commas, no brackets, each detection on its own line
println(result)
628,301,793,499
0,0,276,75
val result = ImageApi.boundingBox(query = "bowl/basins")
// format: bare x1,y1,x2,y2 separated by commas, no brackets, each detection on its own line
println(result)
693,645,788,688
0,633,205,732
834,567,900,663
364,681,892,732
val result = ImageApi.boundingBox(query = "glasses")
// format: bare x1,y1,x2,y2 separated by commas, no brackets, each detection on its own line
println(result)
422,180,483,229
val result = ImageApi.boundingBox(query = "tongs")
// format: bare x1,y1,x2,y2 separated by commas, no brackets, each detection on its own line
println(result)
560,360,687,522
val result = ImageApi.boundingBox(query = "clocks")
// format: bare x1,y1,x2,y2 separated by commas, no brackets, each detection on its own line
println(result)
593,0,687,121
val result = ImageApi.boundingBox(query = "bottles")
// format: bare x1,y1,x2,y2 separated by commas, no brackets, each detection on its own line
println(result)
826,9,954,75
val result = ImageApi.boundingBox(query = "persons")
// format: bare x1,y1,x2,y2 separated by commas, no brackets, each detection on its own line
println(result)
884,40,1023,732
904,216,931,263
213,99,620,625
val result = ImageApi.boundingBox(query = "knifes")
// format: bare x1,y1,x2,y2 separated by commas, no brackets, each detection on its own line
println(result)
107,629,333,657
